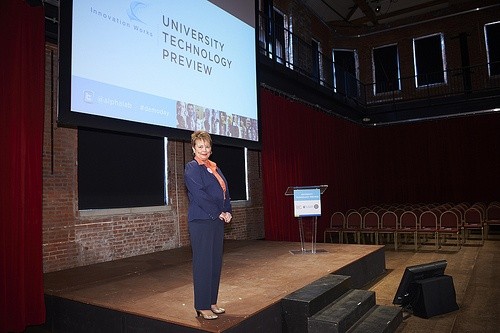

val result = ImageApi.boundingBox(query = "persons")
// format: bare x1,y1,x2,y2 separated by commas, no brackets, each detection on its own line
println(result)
184,131,232,320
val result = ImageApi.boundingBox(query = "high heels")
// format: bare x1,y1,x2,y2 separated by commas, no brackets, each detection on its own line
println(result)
211,306,225,314
196,310,218,319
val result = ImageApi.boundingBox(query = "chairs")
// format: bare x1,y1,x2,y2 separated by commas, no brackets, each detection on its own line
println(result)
324,201,500,252
